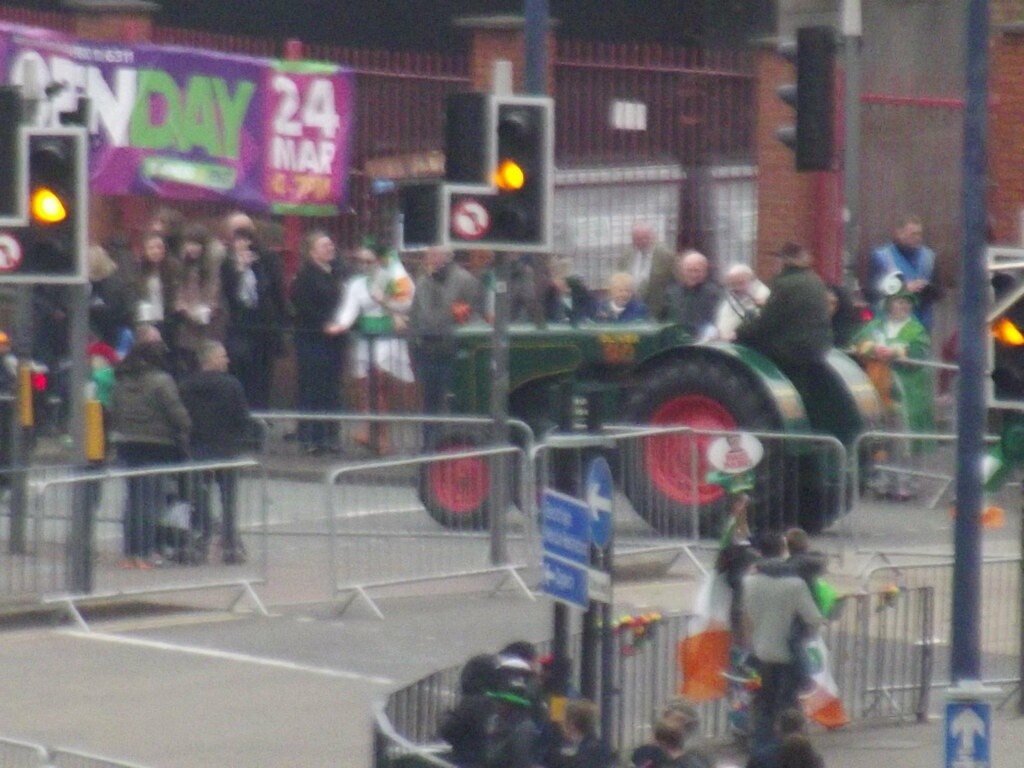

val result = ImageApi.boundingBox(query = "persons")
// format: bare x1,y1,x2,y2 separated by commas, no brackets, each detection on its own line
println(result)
289,211,1023,501
439,639,830,768
1,207,287,567
714,526,827,759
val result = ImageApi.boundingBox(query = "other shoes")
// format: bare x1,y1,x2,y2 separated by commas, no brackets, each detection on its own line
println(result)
328,445,347,461
126,554,160,568
309,447,326,461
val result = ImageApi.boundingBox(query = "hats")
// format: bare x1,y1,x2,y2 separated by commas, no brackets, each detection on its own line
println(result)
882,273,920,308
773,241,814,268
185,222,212,247
227,213,255,238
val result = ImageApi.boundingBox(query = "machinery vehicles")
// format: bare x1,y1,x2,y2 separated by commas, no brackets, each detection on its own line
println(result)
416,310,887,542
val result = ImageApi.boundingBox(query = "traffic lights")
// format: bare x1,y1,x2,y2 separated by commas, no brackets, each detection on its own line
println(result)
775,25,836,169
18,127,90,284
487,100,556,251
990,268,1024,406
